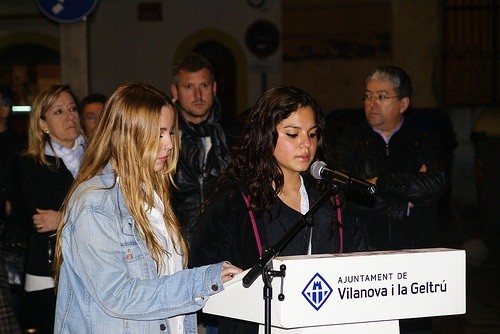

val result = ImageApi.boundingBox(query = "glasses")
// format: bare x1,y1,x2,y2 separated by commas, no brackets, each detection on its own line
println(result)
364,92,398,103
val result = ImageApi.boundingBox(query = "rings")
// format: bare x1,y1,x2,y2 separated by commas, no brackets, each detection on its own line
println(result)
37,224,40,229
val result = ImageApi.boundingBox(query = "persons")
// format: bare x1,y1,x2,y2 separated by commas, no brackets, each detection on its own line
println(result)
0,86,108,334
166,56,231,253
54,83,243,334
189,85,353,334
334,65,456,253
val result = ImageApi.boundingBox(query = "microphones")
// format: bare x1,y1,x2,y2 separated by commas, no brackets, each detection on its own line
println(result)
310,161,375,195
